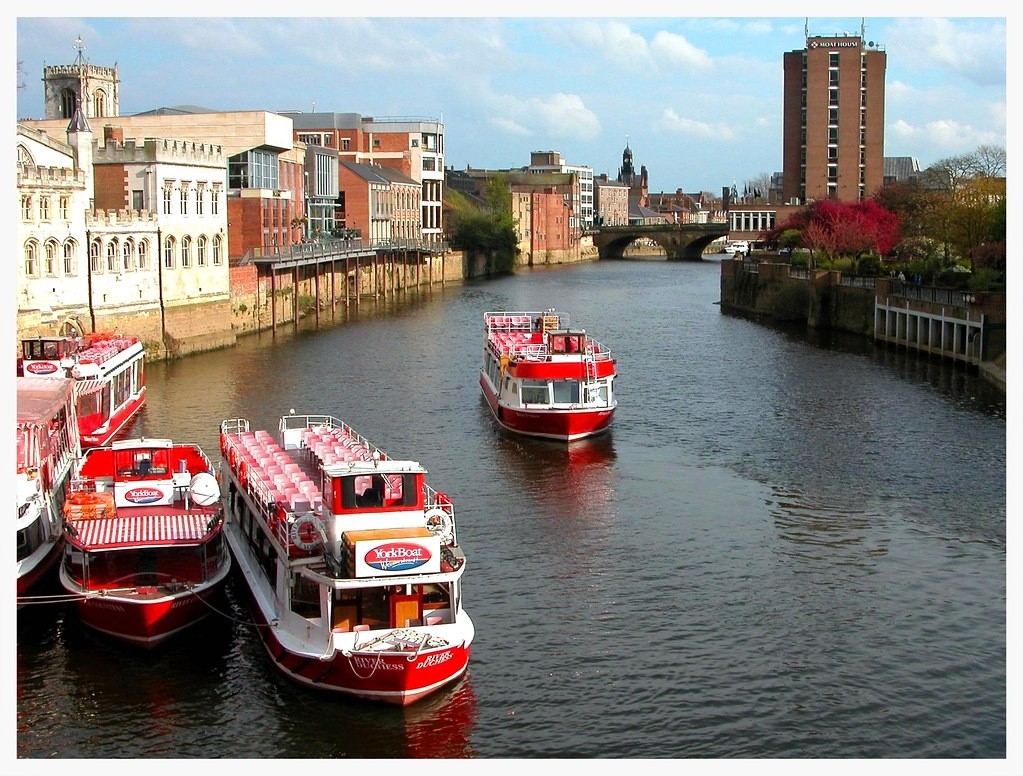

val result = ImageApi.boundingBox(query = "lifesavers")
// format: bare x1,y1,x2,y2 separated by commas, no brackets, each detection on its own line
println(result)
424,508,452,542
290,514,327,550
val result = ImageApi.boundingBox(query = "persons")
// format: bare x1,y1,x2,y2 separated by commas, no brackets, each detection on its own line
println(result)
889,268,922,286
294,235,308,245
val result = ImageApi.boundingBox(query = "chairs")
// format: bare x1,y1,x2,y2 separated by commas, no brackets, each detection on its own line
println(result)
222,423,404,540
45,332,137,364
484,315,598,357
426,615,442,625
352,624,368,631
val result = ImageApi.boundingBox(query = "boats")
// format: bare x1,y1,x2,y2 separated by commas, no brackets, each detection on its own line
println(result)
16,314,149,454
723,242,750,254
17,373,84,615
59,435,233,649
478,306,619,444
216,409,477,708
